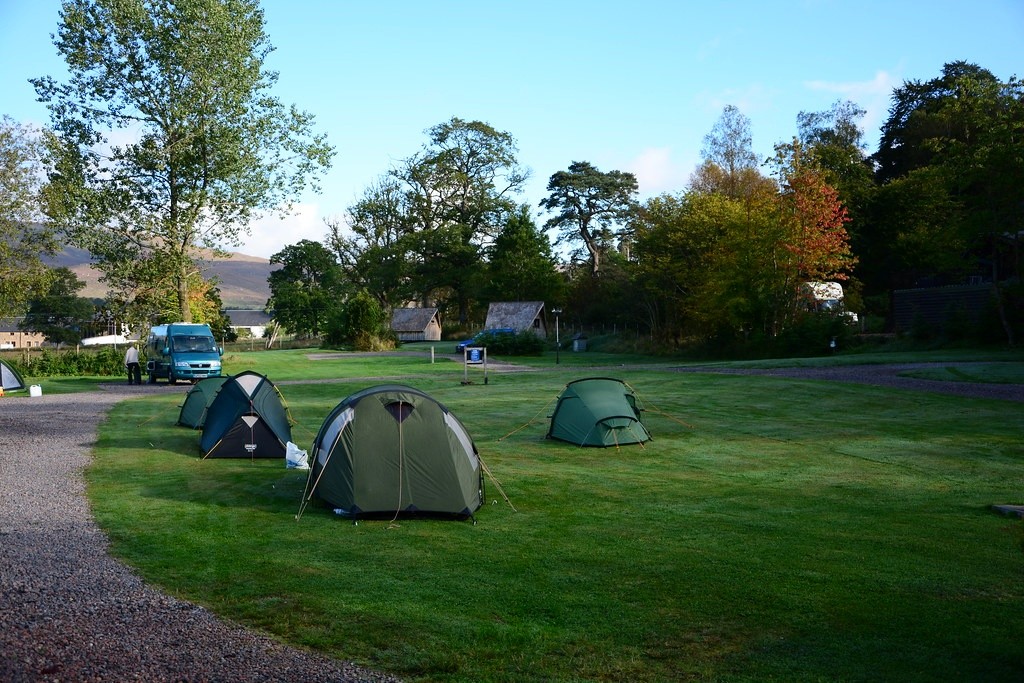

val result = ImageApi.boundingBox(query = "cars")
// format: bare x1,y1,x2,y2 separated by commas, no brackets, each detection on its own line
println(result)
456,328,515,354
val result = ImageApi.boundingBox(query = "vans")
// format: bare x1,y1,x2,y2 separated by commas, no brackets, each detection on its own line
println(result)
143,322,223,385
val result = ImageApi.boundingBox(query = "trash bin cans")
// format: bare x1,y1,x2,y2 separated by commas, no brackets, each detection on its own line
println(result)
572,332,588,353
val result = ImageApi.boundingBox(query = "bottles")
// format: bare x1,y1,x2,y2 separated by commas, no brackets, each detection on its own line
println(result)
334,508,350,515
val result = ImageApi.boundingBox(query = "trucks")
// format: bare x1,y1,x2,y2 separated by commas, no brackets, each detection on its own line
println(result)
793,282,858,326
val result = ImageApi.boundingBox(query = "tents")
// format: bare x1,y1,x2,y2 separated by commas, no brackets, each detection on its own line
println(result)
199,370,293,460
308,384,487,519
0,356,28,394
178,375,230,429
546,377,652,448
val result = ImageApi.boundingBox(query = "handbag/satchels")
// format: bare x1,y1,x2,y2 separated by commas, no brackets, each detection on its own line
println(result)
286,441,310,471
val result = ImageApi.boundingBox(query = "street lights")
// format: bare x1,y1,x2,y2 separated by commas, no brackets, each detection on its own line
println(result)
552,307,563,364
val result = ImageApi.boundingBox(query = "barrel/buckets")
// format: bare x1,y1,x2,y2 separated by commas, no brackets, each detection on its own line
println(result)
30,384,42,397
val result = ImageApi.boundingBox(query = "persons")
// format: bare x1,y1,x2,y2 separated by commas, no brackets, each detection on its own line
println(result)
123,341,143,385
